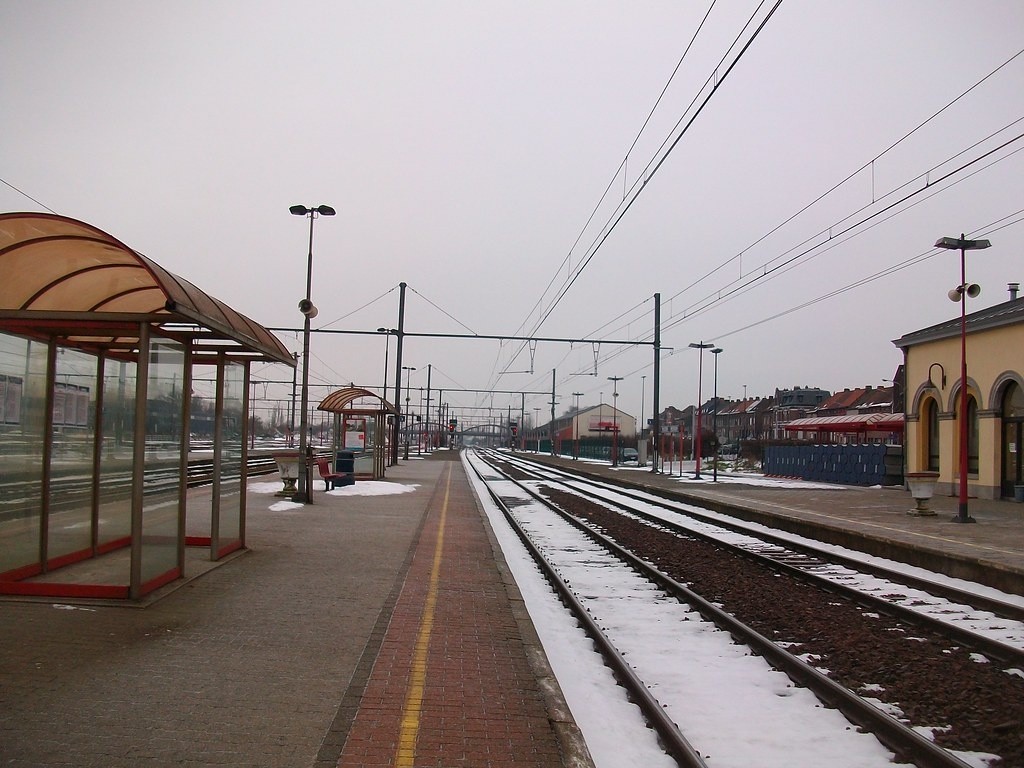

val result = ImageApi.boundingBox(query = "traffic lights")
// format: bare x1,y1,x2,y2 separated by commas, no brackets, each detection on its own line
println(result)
511,427,517,436
449,424,454,432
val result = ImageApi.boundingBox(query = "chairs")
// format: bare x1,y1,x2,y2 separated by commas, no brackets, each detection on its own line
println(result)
316,458,346,490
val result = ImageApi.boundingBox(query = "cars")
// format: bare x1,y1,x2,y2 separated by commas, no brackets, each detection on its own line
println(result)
718,443,738,455
620,447,638,462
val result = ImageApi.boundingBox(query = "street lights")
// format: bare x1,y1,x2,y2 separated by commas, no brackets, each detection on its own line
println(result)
709,348,724,482
606,375,624,468
934,233,991,523
287,204,337,506
689,340,715,480
533,407,541,454
402,366,417,460
637,375,647,467
377,327,398,471
572,391,585,460
